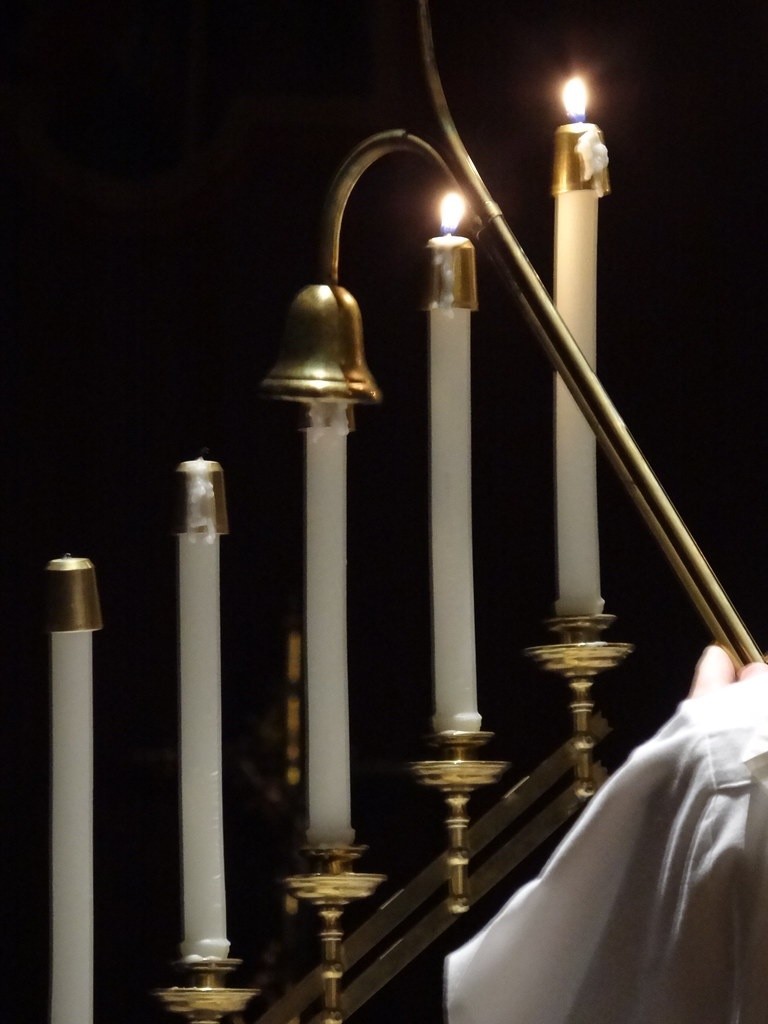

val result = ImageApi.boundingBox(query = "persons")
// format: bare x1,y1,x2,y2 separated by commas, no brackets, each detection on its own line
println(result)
689,643,767,692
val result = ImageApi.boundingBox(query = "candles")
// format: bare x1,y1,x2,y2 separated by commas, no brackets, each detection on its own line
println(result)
38,552,102,1023
418,190,482,735
297,400,372,874
172,459,231,961
550,73,614,618
